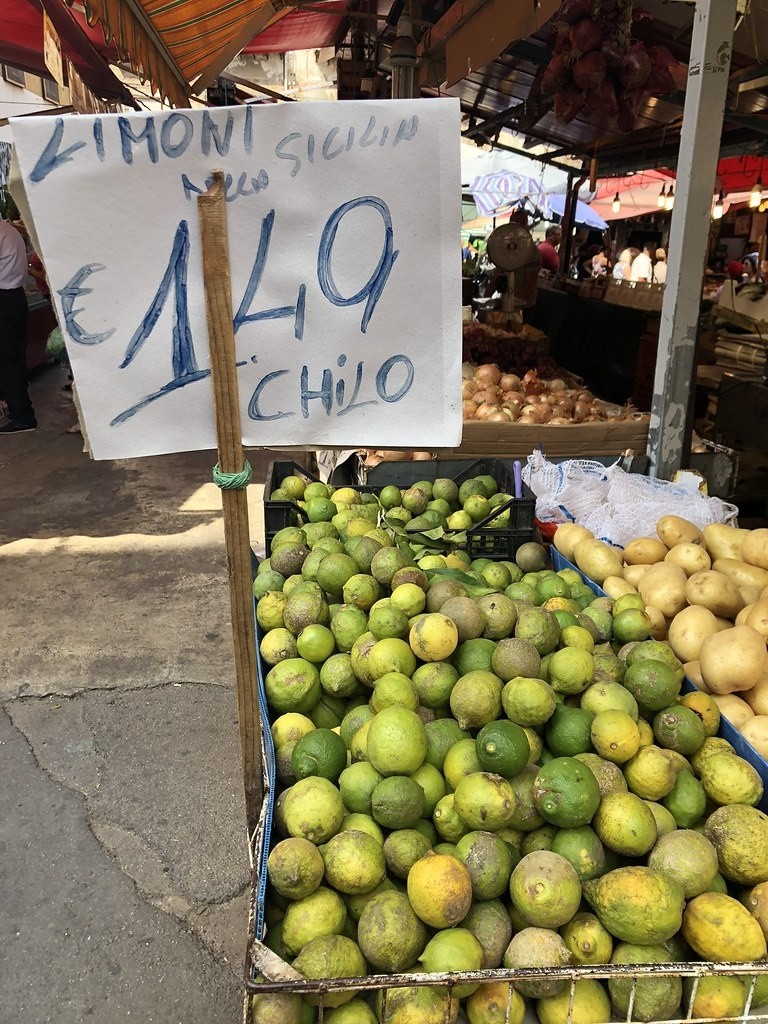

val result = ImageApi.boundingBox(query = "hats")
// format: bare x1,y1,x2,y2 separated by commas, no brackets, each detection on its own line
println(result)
643,242,657,249
725,261,744,277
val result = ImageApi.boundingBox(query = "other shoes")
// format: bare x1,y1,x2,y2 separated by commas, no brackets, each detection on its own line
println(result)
61,383,73,391
6,413,14,420
0,421,37,434
66,422,82,434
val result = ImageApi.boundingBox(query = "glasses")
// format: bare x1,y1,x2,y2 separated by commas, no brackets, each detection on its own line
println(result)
743,262,748,265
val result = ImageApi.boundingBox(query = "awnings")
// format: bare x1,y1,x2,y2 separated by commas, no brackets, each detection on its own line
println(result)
0,0,355,110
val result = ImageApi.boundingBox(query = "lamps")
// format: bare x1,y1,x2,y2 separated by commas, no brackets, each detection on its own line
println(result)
657,183,667,207
389,10,418,98
613,177,621,212
712,189,725,219
749,155,764,207
665,170,675,210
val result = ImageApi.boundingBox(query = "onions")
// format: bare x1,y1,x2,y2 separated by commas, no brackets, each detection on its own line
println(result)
360,361,652,466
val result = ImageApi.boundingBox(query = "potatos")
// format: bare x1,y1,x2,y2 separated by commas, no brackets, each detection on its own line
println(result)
552,514,768,768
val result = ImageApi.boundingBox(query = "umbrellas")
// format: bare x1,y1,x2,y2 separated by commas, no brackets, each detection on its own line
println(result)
471,169,552,222
548,194,609,229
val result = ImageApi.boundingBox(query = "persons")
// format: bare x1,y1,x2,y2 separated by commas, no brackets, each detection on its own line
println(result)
536,225,667,290
461,239,479,262
0,220,80,434
716,253,768,334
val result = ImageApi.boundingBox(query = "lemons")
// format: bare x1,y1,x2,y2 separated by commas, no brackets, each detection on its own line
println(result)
251,470,677,662
243,656,768,1024
